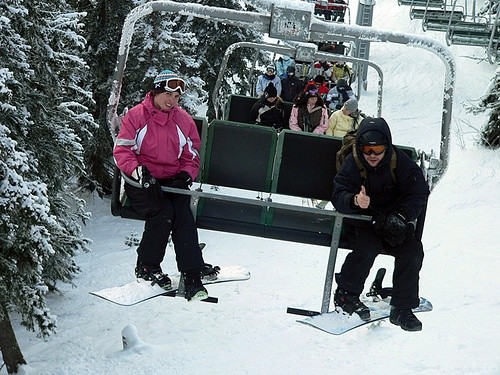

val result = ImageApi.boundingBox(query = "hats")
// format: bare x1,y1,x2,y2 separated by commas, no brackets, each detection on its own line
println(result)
337,79,346,87
152,69,179,96
359,131,388,145
344,98,358,113
304,84,318,97
266,63,276,71
264,83,277,99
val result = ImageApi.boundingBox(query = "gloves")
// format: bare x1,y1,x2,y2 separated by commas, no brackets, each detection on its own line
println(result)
371,212,407,248
168,172,193,190
382,209,409,240
131,165,164,201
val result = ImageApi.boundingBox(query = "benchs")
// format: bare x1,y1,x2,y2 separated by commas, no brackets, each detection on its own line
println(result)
108,111,427,254
227,95,367,121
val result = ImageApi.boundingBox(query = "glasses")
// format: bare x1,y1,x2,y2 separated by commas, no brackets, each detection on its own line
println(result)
164,78,186,96
267,69,274,73
358,143,386,157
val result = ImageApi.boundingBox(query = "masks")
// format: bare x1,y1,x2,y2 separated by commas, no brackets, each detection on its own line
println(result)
339,87,344,91
288,72,293,76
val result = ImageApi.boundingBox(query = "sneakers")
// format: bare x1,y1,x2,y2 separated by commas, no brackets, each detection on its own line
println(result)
389,306,423,331
334,287,370,321
178,272,208,303
135,262,171,289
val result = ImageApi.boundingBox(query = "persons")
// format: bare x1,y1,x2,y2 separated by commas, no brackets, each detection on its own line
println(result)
277,55,293,78
255,63,283,99
279,65,303,102
301,75,330,105
328,99,364,136
330,61,352,83
308,60,330,78
317,41,347,54
248,82,284,130
293,61,313,79
331,117,429,332
113,69,207,302
289,84,329,137
327,80,356,110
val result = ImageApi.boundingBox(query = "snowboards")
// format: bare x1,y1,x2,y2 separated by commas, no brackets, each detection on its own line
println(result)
296,288,433,335
89,265,252,306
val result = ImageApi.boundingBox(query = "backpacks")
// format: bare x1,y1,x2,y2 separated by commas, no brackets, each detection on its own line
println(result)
335,130,398,183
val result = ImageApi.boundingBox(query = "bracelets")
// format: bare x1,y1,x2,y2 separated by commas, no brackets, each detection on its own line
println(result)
356,194,358,204
